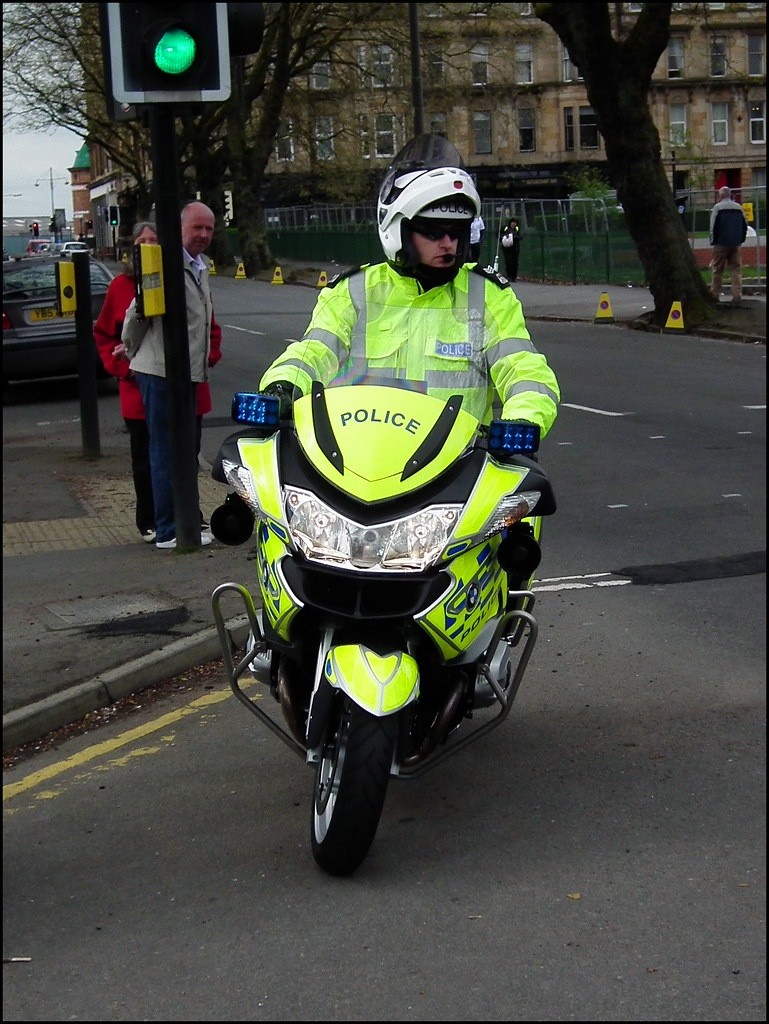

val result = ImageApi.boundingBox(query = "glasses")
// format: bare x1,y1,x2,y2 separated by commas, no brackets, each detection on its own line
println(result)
408,222,460,242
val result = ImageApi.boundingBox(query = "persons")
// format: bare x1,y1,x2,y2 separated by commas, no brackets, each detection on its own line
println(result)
258,134,562,462
470,216,485,262
78,233,85,242
112,202,216,548
709,185,747,302
501,217,523,283
94,222,223,542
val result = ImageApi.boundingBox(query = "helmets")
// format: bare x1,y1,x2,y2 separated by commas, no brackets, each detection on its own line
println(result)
376,165,481,268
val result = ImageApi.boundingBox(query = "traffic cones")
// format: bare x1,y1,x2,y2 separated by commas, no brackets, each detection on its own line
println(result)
316,271,327,289
594,290,615,324
270,266,284,285
209,259,217,275
235,263,246,279
662,298,686,334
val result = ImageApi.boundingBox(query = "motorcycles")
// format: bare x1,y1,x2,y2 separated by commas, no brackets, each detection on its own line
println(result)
210,294,557,879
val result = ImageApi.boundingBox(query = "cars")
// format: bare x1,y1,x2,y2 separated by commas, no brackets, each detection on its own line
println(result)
0,254,115,403
26,238,91,259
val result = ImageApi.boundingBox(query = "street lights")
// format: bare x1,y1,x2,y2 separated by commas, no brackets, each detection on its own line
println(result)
34,166,70,243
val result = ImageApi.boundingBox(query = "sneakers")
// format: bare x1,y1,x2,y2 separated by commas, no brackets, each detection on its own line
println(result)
152,533,218,548
140,529,156,544
200,520,212,532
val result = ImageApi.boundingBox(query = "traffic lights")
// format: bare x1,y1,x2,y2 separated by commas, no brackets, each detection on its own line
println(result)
33,222,39,236
225,1,265,57
88,220,93,229
108,205,120,226
107,1,231,105
29,223,33,232
223,191,234,220
